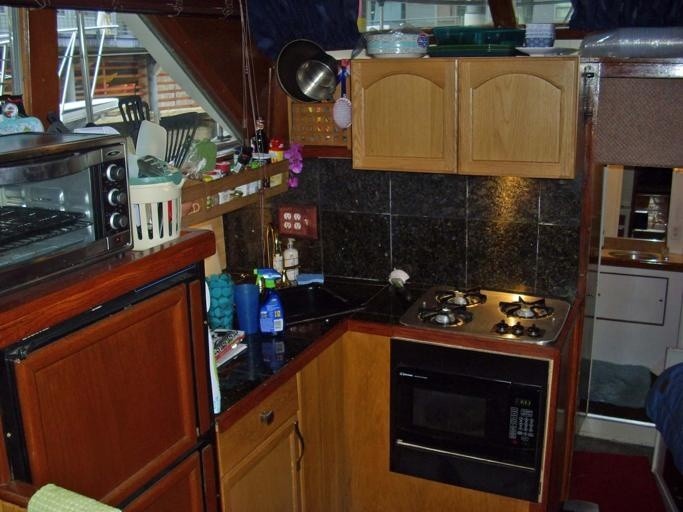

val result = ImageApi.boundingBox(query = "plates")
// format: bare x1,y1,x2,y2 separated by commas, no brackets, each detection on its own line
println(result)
515,45,576,56
364,31,427,58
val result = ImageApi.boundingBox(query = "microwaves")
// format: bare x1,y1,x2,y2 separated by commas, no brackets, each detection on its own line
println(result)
0,131,132,299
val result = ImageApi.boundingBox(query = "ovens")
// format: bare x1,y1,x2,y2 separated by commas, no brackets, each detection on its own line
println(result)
387,339,552,504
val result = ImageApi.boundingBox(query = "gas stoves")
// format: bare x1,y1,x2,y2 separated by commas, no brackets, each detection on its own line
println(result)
400,285,570,346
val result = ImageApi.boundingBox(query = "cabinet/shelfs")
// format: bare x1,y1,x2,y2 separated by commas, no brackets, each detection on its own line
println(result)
213,370,306,512
349,56,598,180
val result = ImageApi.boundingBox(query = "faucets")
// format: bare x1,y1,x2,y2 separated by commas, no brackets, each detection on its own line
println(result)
651,219,668,252
280,264,305,286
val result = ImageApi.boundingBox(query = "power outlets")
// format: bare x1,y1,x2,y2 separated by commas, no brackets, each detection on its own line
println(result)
276,202,319,242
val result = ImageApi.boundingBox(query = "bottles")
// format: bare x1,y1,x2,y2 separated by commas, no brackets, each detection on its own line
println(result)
232,118,269,198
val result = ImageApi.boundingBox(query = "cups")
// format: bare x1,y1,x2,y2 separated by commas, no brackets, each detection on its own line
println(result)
234,283,258,336
199,274,234,332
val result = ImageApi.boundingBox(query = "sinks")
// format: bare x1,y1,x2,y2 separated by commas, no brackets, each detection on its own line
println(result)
260,284,353,320
608,250,660,262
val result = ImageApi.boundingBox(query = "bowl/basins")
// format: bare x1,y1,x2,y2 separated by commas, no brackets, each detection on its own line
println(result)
522,24,555,46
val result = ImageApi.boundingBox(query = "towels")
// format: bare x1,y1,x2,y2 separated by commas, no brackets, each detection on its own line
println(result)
26,483,126,512
